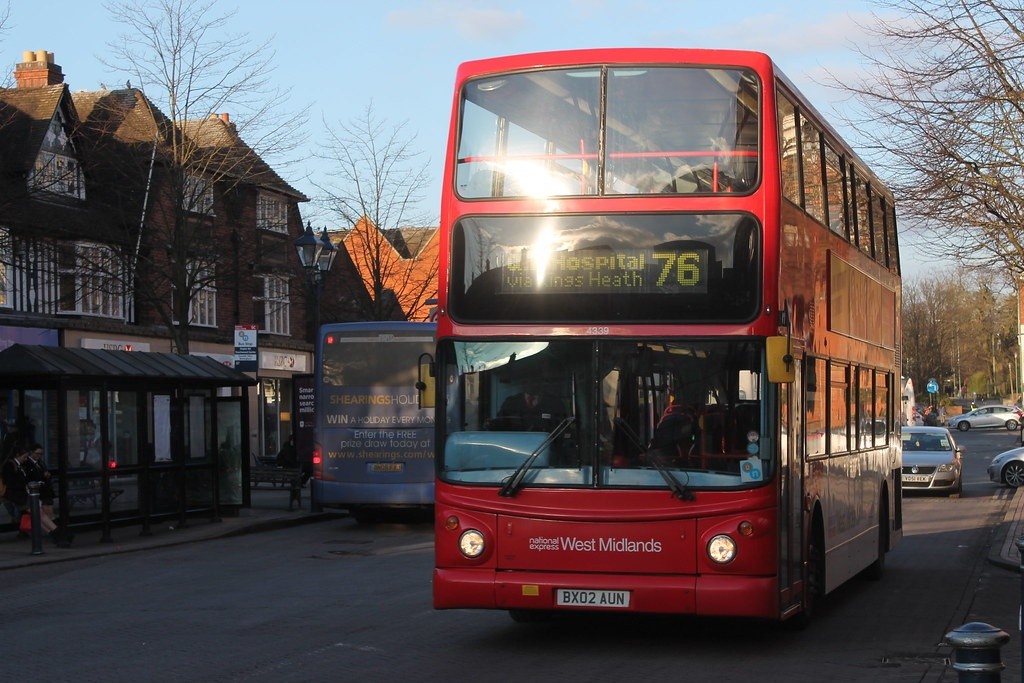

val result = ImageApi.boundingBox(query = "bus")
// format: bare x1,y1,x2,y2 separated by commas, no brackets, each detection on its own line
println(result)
415,48,903,630
311,321,435,525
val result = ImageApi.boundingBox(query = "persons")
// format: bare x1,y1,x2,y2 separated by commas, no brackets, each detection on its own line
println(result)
277,435,310,488
483,368,566,447
945,384,967,399
0,442,57,542
84,422,100,462
912,405,946,426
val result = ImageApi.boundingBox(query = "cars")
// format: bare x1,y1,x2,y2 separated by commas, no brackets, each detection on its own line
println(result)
901,426,967,498
987,447,1024,487
947,405,1024,431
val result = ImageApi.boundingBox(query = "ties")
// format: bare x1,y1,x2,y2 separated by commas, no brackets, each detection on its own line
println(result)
527,395,536,406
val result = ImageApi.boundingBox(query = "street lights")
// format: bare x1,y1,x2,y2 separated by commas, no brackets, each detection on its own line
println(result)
935,318,961,397
293,220,338,330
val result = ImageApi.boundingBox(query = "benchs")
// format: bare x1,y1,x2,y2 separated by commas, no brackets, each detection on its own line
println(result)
55,487,124,510
249,466,302,510
253,454,288,487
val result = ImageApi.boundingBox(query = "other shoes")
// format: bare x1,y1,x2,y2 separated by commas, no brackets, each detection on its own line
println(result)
48,529,75,547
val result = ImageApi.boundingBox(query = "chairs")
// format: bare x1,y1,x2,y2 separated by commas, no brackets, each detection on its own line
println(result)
656,404,760,473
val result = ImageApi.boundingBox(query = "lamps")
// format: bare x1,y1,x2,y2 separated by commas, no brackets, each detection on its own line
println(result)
293,219,340,291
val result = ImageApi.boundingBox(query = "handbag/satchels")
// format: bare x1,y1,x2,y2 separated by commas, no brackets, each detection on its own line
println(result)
19,513,32,532
